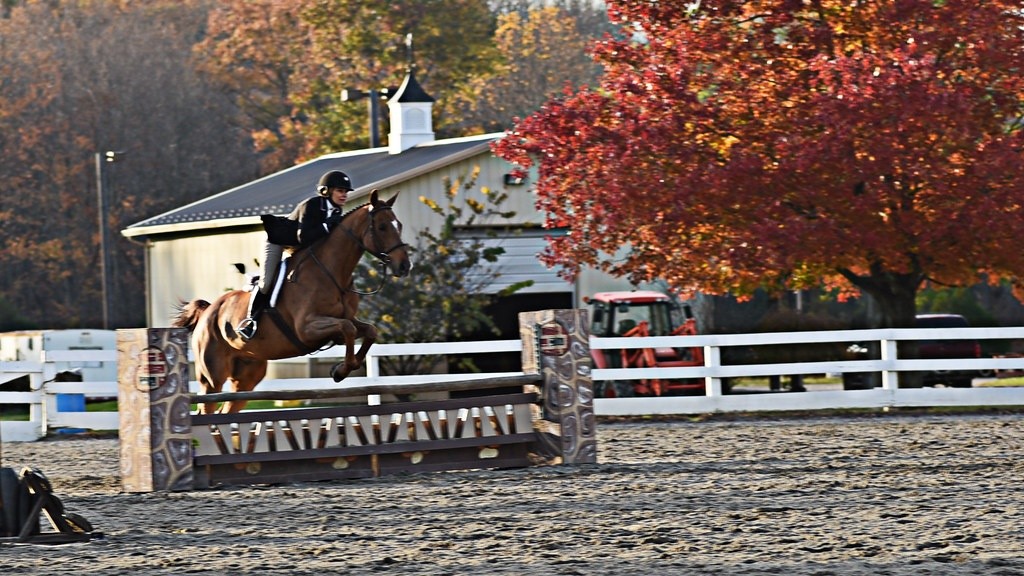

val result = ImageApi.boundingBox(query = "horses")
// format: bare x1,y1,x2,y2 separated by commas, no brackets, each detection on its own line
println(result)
172,188,410,418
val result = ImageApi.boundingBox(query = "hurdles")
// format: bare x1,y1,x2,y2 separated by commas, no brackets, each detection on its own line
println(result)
111,306,601,494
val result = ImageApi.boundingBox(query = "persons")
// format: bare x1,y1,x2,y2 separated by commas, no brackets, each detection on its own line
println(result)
240,171,354,338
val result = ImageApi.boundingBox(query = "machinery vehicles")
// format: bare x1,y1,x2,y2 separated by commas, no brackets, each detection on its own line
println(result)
583,290,706,398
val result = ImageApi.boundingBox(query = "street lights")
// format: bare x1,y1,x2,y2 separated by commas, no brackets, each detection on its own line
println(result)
94,151,127,332
340,85,397,149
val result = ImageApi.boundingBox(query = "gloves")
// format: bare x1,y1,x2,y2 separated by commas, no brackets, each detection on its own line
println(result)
322,214,342,233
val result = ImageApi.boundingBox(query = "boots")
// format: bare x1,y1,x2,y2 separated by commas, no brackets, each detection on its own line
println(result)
239,285,269,340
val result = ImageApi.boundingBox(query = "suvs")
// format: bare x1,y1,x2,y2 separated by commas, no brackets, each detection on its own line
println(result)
842,313,981,390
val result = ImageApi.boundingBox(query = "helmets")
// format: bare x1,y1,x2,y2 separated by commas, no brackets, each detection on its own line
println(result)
319,171,354,191
